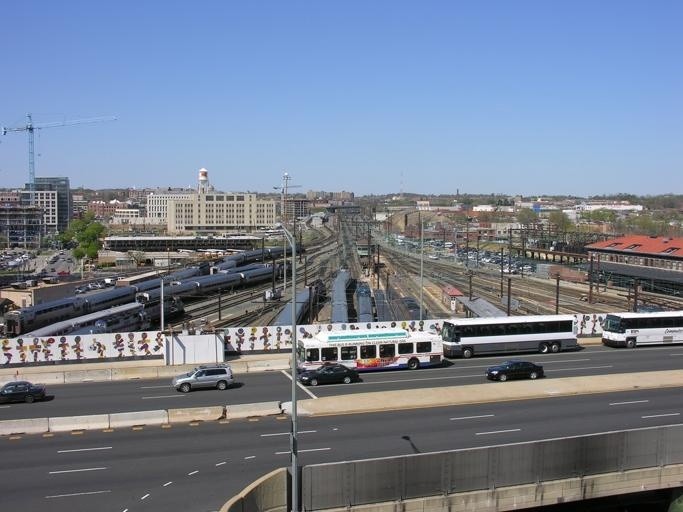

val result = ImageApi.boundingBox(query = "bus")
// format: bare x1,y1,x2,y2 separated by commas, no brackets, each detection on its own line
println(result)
602,312,683,348
442,314,577,359
296,327,445,372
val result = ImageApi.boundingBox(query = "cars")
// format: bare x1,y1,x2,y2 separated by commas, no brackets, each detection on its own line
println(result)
397,234,536,273
299,362,360,385
1,382,45,403
485,360,544,381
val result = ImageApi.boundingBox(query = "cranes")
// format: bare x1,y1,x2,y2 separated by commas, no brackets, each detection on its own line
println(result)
2,113,119,207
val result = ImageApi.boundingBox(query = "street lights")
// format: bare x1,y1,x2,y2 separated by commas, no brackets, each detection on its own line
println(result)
273,222,298,512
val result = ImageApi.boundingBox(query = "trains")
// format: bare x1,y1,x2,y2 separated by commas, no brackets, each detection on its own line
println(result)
271,271,427,325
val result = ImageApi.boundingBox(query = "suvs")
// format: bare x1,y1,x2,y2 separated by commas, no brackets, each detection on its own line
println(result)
172,364,233,392
